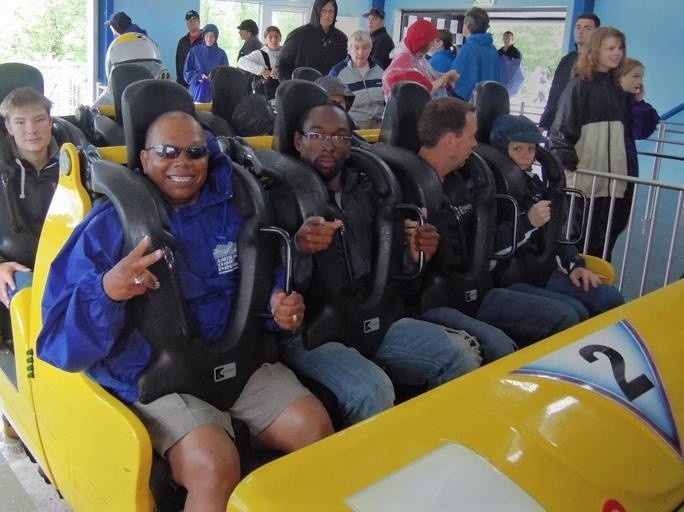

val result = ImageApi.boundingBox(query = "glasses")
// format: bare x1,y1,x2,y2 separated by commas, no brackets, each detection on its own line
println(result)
301,132,352,148
146,145,207,159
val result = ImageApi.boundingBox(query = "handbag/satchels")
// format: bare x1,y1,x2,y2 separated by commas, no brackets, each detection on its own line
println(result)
252,73,280,99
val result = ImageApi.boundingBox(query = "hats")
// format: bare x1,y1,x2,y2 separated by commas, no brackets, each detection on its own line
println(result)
362,8,385,19
104,20,110,25
237,19,256,32
510,131,549,143
185,10,198,20
